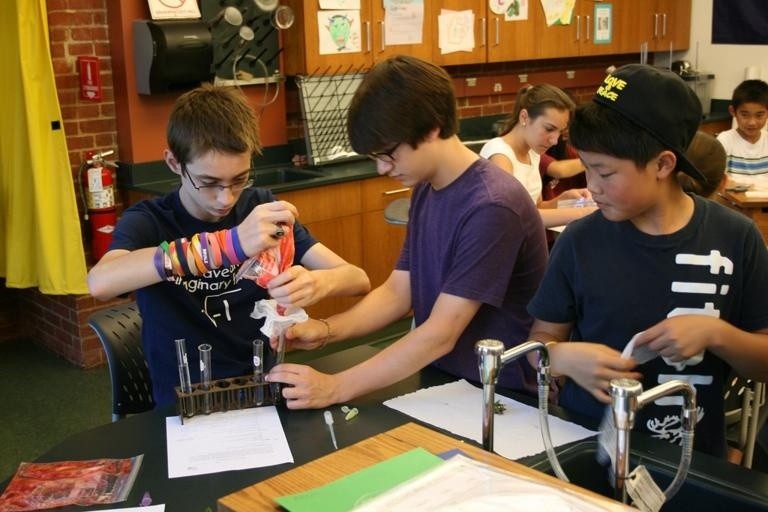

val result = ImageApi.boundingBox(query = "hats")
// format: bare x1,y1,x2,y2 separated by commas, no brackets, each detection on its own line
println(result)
593,62,706,189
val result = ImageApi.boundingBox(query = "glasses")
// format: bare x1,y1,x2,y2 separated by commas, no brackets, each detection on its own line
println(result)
181,165,257,195
365,143,400,162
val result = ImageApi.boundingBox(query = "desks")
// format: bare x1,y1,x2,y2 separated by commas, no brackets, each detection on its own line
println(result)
721,180,768,247
0,409,768,512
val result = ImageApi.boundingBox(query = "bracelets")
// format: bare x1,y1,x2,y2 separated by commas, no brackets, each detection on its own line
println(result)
154,226,251,283
534,339,564,382
318,318,332,353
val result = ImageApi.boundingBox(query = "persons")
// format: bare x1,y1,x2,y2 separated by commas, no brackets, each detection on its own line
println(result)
478,82,600,229
525,62,768,458
85,80,373,411
677,133,750,465
263,55,556,410
714,79,768,199
539,116,586,201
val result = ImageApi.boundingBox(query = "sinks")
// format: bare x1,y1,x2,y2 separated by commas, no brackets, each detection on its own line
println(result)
527,440,767,512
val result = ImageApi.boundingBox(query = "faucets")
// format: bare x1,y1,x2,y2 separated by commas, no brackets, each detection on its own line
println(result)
609,374,700,502
476,336,552,453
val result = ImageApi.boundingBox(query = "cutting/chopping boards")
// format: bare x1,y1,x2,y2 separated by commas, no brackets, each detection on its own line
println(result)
211,422,642,511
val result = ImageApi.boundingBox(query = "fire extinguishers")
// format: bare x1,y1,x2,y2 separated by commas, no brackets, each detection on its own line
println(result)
78,149,119,266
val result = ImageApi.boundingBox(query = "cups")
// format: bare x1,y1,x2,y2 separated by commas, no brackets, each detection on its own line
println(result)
211,0,297,63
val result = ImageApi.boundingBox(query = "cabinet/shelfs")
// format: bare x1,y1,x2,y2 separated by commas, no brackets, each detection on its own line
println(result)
431,0,540,67
625,0,692,53
281,0,432,76
275,177,414,318
540,0,626,59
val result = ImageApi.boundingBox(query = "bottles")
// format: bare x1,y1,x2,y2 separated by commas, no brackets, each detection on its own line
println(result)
275,334,287,362
198,342,212,413
174,338,196,417
251,339,265,406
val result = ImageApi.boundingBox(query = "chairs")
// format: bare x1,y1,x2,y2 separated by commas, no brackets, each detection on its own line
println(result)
725,371,768,469
87,301,152,421
